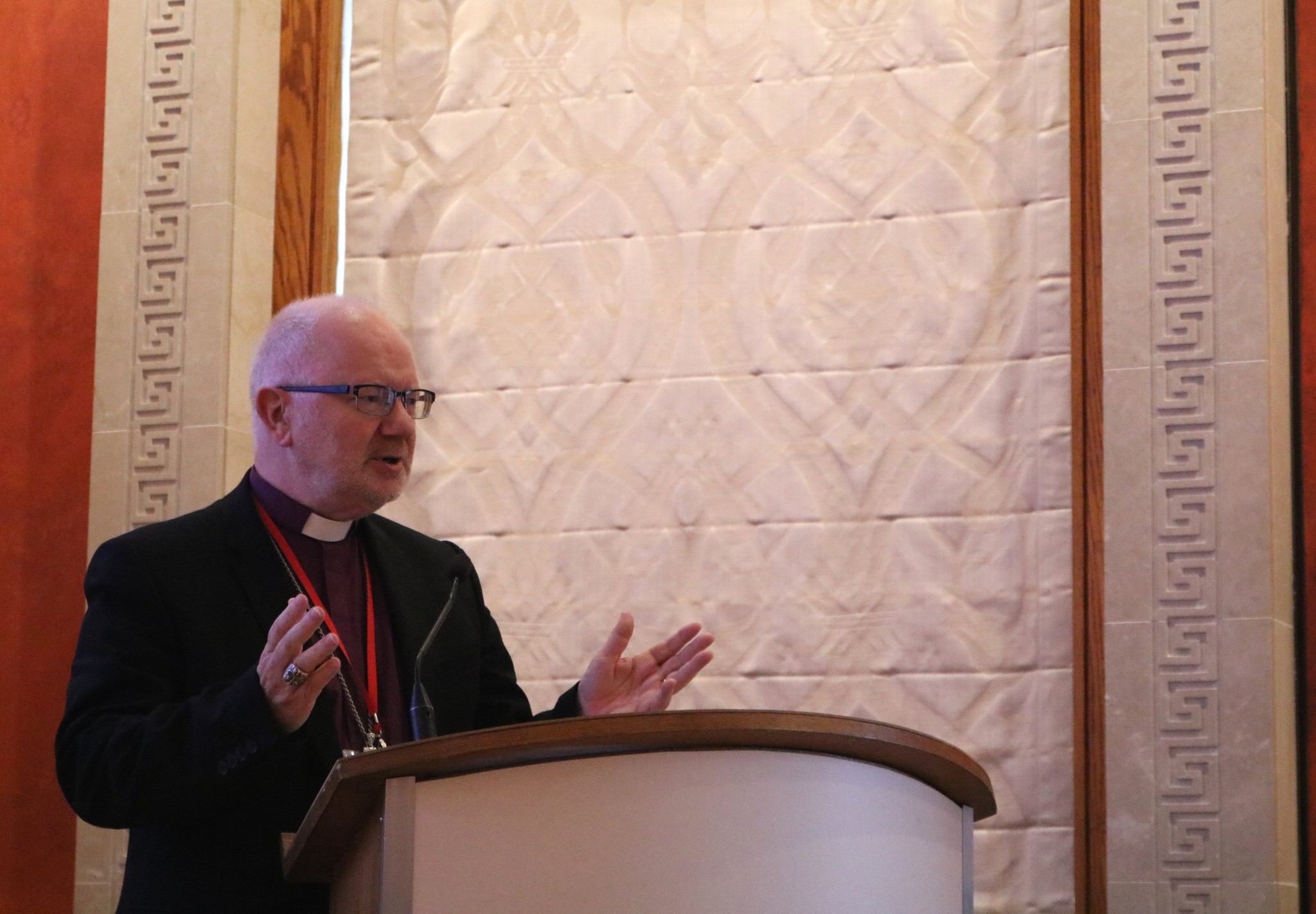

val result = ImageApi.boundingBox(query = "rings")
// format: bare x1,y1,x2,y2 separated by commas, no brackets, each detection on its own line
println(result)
283,662,310,690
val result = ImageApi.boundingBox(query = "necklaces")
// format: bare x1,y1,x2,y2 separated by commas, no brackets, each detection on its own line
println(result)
266,529,378,751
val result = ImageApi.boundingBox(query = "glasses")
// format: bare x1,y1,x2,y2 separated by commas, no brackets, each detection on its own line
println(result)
277,384,435,419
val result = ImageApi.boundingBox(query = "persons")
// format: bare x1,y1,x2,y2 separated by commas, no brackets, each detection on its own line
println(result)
54,293,715,914
